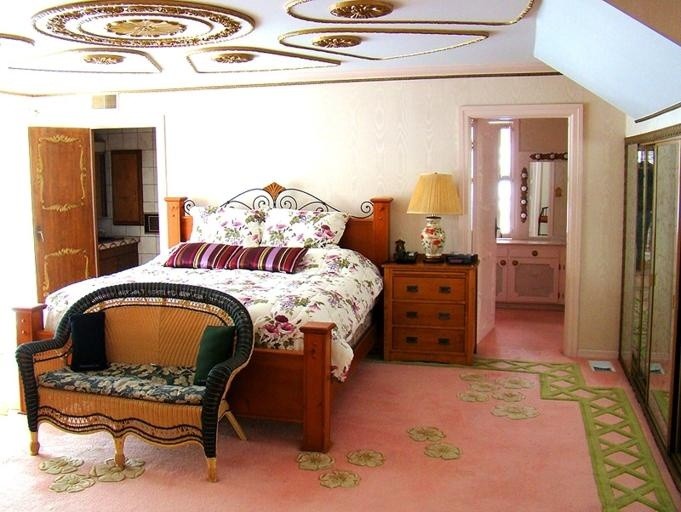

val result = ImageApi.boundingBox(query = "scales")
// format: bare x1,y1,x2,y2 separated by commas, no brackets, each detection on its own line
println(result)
646,362,664,375
588,360,617,373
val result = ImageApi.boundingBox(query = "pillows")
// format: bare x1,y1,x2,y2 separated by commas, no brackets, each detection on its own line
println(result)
261,207,352,248
192,322,238,383
164,240,243,275
188,205,266,247
226,245,310,275
67,309,110,371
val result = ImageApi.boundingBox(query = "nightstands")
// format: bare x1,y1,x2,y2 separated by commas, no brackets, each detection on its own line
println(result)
382,261,478,364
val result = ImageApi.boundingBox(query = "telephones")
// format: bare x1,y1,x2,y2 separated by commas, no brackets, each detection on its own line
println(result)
393,239,418,264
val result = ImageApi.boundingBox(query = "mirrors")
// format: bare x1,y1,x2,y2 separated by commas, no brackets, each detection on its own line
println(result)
528,161,570,240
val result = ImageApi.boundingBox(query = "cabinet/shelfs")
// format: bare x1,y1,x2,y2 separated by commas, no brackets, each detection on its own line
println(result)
493,243,561,303
100,242,141,276
561,246,567,303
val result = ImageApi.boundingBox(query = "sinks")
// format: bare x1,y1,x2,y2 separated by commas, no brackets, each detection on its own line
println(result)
98,236,125,245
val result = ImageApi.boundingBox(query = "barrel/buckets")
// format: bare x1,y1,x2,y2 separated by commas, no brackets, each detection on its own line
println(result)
96,217,110,236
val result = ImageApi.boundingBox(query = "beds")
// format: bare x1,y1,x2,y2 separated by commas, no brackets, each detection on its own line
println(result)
14,185,394,450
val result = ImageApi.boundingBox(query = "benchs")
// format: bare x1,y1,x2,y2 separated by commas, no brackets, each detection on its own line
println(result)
15,282,257,483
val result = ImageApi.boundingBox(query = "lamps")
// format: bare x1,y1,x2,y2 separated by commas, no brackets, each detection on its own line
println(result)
406,172,464,258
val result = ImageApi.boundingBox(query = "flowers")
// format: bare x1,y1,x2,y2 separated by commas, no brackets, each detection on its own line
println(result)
38,451,148,497
298,423,461,491
458,367,538,424
